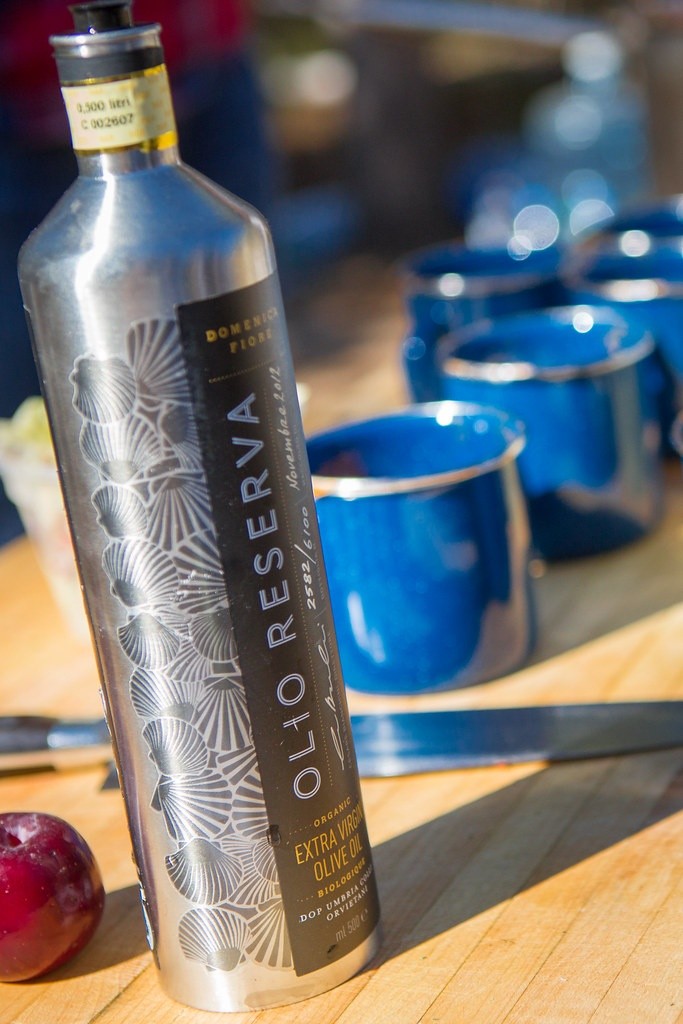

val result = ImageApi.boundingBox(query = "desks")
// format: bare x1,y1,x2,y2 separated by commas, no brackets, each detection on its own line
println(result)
0,222,683,1024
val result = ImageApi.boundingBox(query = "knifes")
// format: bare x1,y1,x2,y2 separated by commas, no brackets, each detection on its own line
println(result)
1,700,682,789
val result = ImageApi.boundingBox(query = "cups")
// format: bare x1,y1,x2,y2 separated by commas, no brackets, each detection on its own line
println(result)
305,401,539,695
399,198,683,561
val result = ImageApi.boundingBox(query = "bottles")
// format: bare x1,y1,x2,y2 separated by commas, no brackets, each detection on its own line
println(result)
16,0,386,1010
528,34,650,199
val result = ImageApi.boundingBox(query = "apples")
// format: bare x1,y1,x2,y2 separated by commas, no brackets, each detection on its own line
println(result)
0,812,104,983
